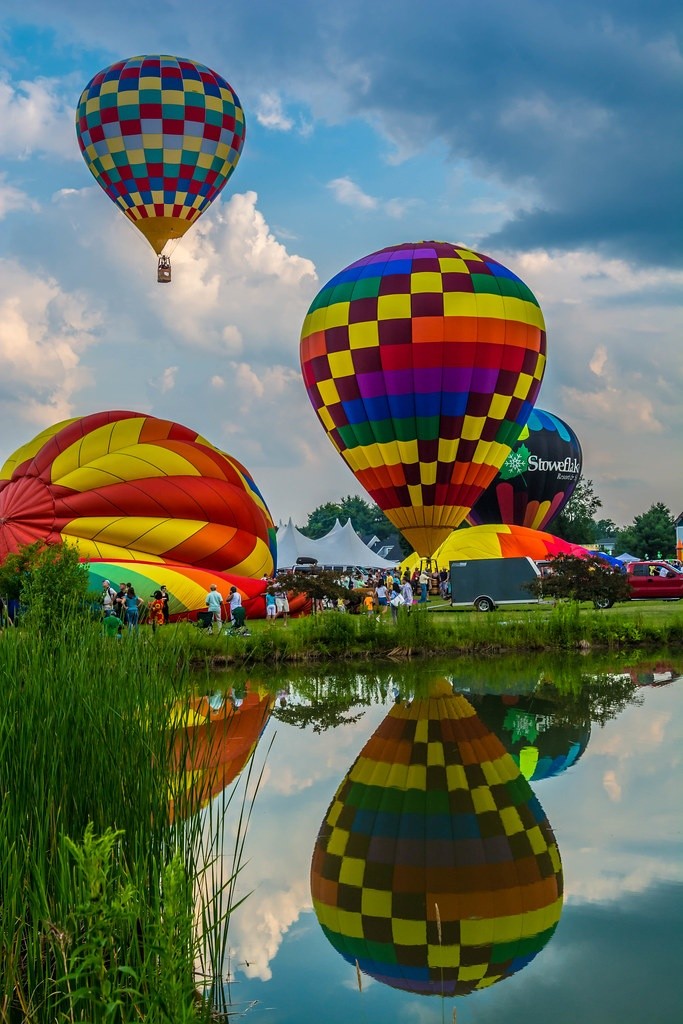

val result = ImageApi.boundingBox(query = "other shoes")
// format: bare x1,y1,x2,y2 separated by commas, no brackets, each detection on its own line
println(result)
272,622,277,626
375,616,387,623
283,623,287,627
208,629,223,635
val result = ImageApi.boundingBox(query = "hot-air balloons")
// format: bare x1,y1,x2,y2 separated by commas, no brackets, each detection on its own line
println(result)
296,239,548,576
311,670,566,997
74,54,247,282
0,410,277,621
457,676,593,783
463,407,583,532
397,523,629,583
120,680,275,832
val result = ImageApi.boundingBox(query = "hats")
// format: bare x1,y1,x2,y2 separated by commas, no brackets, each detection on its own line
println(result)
415,567,419,569
422,571,428,574
443,566,448,569
103,580,110,584
210,584,217,589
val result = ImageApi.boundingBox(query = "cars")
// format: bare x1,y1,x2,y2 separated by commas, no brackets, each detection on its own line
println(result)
536,557,683,609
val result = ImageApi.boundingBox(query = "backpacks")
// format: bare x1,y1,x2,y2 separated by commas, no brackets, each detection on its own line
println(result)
107,587,117,606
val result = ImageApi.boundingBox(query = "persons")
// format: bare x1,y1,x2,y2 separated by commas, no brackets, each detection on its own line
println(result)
114,583,144,634
149,591,164,634
226,587,242,625
608,561,683,576
261,567,450,623
277,684,290,709
205,584,223,635
274,577,290,626
160,585,169,625
265,587,276,626
103,612,123,640
101,579,117,617
204,686,247,714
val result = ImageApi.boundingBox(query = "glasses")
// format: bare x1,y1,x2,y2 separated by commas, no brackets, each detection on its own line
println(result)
162,587,166,590
444,570,447,572
